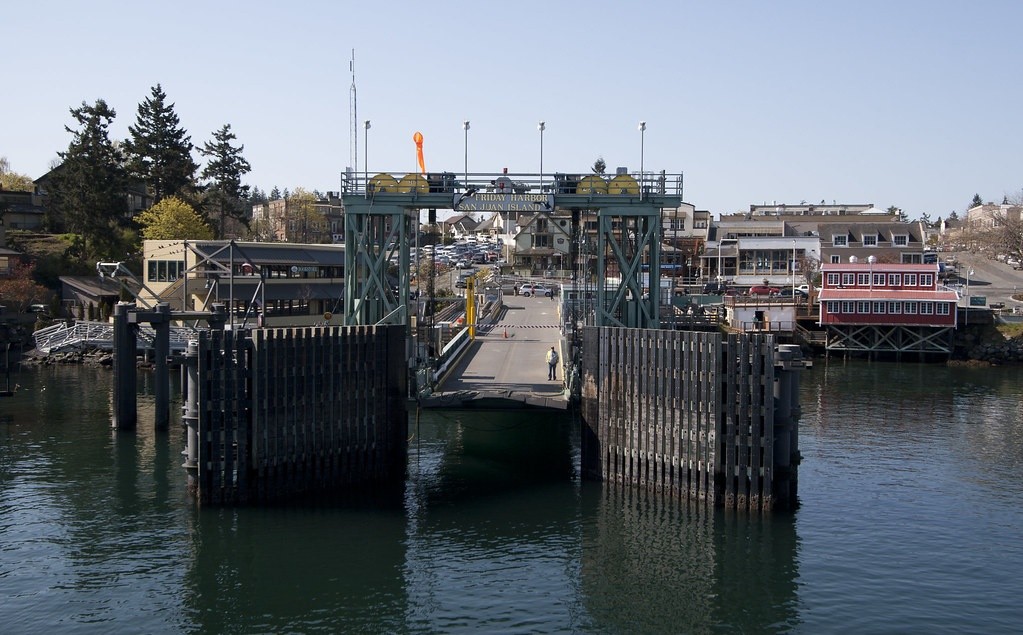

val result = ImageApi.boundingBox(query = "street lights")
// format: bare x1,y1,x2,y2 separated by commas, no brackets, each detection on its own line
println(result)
363,120,371,198
463,120,470,190
639,120,646,201
537,122,545,192
792,239,796,300
553,253,564,305
958,266,965,284
965,266,975,326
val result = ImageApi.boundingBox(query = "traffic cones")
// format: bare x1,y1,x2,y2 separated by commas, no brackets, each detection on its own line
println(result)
502,328,509,338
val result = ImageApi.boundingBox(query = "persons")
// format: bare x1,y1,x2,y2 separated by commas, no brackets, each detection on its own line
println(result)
513,283,517,296
529,284,536,298
546,347,559,381
550,289,555,301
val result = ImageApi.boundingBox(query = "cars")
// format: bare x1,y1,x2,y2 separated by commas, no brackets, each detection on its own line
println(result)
781,288,807,297
796,285,822,295
749,285,779,297
945,255,957,263
924,242,1023,271
410,233,503,272
455,271,473,288
644,286,687,297
723,290,746,298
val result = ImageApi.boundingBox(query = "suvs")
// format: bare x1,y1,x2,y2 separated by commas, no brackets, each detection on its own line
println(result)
924,253,938,264
704,283,730,296
518,284,551,297
